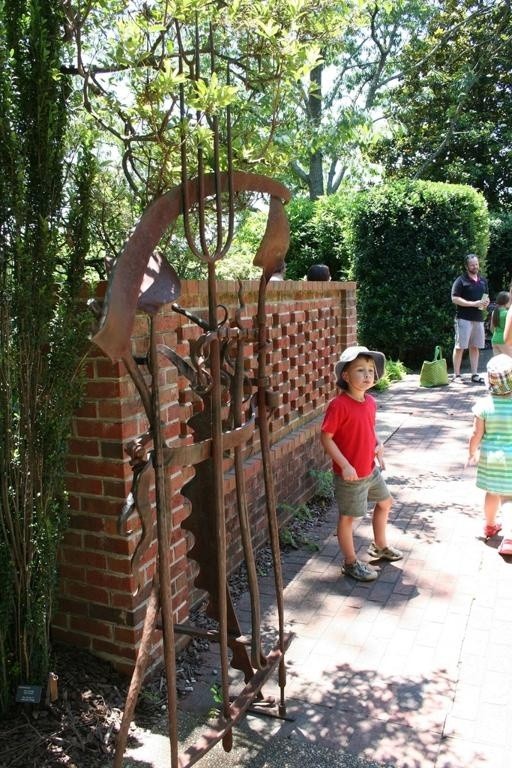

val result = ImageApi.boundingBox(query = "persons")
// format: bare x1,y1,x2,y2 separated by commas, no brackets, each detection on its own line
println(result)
468,353,511,556
269,258,292,282
503,287,512,348
490,290,512,358
450,253,490,385
319,345,404,583
306,264,332,282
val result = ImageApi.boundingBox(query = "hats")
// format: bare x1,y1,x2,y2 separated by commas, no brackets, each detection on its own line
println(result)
486,354,512,395
336,345,385,388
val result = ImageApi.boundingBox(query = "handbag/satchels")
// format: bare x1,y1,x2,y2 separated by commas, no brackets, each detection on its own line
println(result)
419,345,449,388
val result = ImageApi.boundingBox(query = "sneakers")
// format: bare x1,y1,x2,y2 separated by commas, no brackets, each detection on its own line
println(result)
366,541,403,560
341,559,378,581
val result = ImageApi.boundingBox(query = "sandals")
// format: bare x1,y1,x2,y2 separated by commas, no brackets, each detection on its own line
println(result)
451,374,466,384
471,374,484,384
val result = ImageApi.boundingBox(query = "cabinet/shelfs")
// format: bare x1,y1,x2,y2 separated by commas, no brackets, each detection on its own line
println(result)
84,171,292,768
